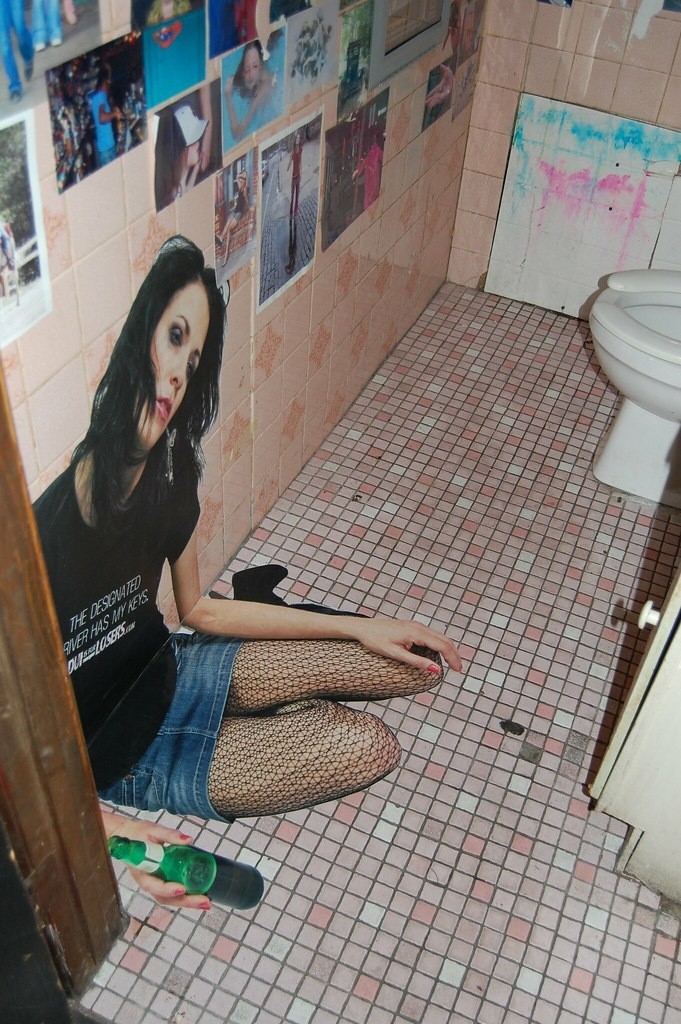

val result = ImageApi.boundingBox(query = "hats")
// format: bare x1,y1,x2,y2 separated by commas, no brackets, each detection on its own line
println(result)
173,105,210,147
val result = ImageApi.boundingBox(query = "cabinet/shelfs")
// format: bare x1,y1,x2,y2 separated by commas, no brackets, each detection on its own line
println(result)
586,560,681,907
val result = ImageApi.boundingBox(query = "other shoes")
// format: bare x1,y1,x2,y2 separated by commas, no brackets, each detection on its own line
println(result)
51,36,62,46
222,255,229,267
215,236,222,246
11,91,21,101
35,43,46,52
24,65,33,79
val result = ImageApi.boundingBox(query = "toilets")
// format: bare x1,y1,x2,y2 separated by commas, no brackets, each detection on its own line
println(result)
589,269,681,511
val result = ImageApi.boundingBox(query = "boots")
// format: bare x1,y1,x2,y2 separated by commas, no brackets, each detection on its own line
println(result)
208,564,369,619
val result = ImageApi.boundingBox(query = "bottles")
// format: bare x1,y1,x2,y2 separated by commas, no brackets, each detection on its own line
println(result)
105,831,265,912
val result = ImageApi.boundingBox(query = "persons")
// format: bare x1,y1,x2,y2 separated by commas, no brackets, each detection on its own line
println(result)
224,40,275,141
91,79,122,168
424,62,455,109
30,235,464,913
0,218,19,301
285,134,305,218
215,172,251,269
1,0,79,104
154,86,214,211
